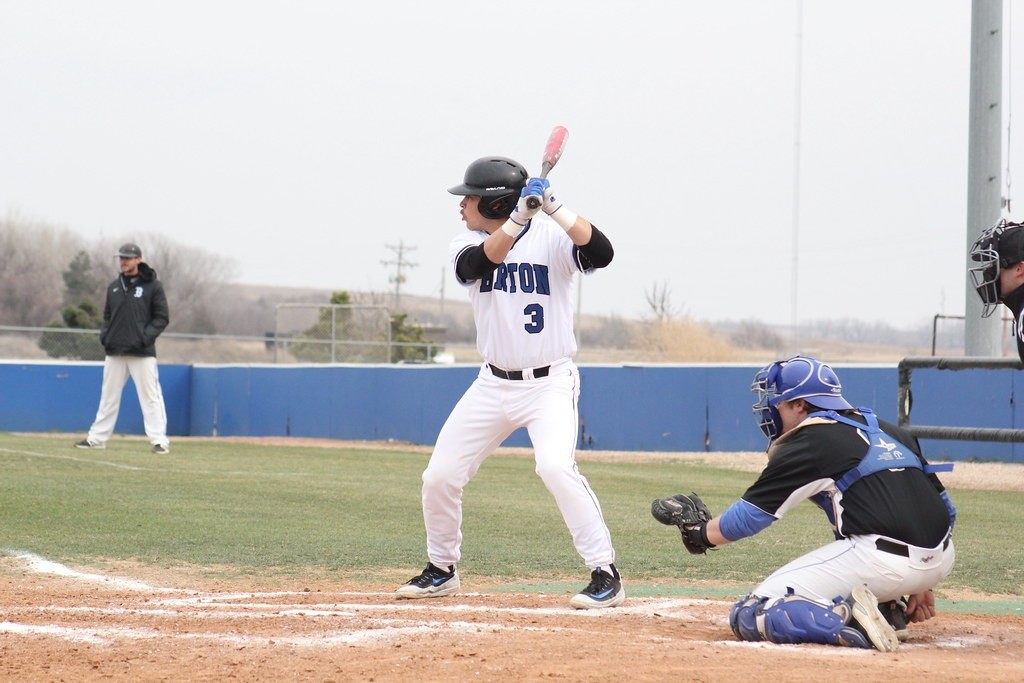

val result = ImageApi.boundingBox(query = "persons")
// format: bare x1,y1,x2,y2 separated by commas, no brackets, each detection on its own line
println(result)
396,156,625,609
968,216,1024,367
74,243,169,455
651,354,957,653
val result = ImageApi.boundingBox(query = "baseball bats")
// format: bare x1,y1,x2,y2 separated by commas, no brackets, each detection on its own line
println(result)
527,125,569,209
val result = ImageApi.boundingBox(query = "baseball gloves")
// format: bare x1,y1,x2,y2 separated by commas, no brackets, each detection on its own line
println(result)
650,492,712,555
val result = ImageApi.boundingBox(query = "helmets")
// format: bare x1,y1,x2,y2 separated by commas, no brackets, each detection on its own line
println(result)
448,155,530,219
775,356,853,409
971,224,1024,266
115,244,142,259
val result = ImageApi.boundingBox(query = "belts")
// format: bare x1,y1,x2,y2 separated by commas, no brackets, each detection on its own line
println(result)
875,535,949,557
488,363,551,380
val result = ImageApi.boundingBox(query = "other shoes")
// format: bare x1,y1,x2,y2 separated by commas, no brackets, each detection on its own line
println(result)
846,587,899,653
154,444,166,455
75,439,106,448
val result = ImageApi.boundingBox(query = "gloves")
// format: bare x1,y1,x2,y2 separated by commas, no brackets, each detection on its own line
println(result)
526,178,563,216
509,175,545,226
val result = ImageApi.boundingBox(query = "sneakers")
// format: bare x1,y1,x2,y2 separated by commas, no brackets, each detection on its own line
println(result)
395,561,460,597
570,562,626,610
